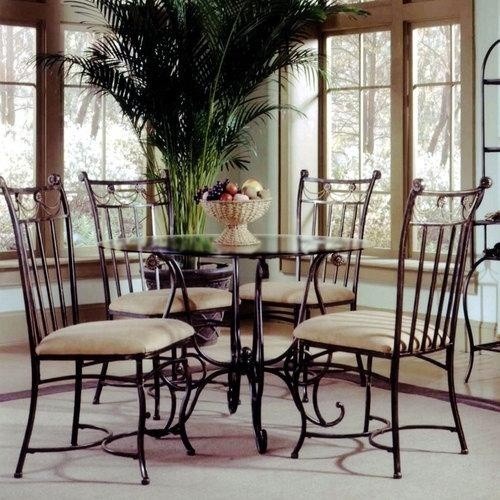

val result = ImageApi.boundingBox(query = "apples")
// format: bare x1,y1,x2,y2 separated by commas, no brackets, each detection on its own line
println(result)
222,178,271,203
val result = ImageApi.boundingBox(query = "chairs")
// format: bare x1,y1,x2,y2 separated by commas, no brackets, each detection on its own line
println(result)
287,176,491,479
79,171,243,421
236,170,381,406
0,173,194,485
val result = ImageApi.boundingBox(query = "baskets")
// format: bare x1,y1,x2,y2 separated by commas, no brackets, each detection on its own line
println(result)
199,199,272,246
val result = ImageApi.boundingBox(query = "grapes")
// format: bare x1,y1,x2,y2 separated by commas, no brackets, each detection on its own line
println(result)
193,178,230,204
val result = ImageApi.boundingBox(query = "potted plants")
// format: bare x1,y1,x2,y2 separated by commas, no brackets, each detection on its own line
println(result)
24,0,371,347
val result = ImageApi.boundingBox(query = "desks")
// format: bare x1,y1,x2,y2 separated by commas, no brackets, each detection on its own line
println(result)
94,235,374,455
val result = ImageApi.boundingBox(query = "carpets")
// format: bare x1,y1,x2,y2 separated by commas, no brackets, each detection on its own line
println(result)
0,360,500,499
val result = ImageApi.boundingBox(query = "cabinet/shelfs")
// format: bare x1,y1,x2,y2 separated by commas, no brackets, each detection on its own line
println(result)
461,40,500,385
